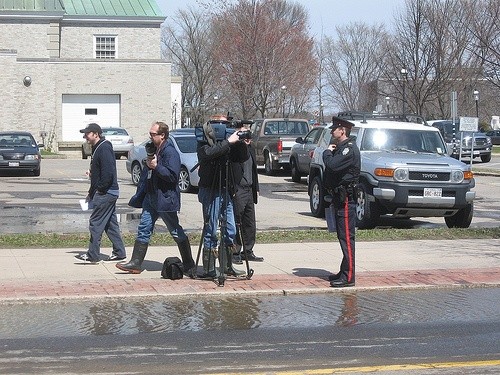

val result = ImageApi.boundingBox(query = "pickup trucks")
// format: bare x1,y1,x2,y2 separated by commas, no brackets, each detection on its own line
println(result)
249,118,311,176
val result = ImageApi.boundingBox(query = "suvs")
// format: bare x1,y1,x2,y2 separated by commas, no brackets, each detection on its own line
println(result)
308,112,476,230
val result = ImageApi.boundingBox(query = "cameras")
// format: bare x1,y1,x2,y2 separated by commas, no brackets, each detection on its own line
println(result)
145,143,157,160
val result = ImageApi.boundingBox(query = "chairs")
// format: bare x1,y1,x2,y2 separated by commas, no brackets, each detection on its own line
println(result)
21,139,29,145
0,139,7,145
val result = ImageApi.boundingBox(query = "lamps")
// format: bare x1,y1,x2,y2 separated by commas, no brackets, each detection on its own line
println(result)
24,76,32,84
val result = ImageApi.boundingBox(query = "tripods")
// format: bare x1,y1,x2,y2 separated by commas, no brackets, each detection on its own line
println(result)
189,154,254,288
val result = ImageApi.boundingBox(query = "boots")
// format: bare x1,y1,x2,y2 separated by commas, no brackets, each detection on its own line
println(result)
219,246,247,277
116,240,148,273
179,238,194,272
191,248,214,280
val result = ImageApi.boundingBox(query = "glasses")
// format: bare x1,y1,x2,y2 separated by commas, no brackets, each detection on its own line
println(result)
149,132,162,136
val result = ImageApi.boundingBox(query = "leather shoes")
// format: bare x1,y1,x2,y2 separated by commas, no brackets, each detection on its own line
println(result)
247,253,264,261
329,274,340,280
331,279,355,288
233,254,243,264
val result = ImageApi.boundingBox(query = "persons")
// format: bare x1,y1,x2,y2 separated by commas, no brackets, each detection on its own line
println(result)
322,116,362,288
116,122,195,273
229,120,264,264
194,115,251,276
74,123,127,263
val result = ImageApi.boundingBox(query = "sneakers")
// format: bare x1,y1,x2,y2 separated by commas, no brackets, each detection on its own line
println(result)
74,252,103,263
106,252,126,261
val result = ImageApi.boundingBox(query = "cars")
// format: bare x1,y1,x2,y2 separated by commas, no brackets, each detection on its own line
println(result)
0,131,44,176
424,121,492,162
289,126,325,182
483,130,500,144
81,127,134,159
126,128,201,193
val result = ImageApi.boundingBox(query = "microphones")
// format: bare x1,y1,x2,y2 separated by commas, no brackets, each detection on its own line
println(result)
236,120,253,124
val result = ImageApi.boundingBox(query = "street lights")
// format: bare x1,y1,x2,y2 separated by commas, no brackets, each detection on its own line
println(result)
282,86,287,118
385,97,390,112
400,68,408,114
473,91,478,117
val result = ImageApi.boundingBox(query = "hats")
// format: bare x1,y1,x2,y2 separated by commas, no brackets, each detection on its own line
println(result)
328,116,355,128
80,123,102,133
